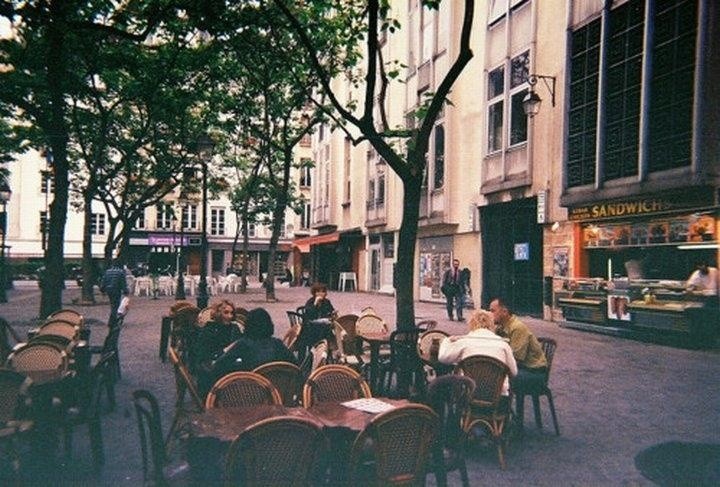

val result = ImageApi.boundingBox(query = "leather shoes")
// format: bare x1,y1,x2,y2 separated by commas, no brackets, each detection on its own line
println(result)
450,317,453,321
458,317,465,321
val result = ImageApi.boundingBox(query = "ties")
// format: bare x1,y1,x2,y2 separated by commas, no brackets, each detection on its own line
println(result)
454,268,457,286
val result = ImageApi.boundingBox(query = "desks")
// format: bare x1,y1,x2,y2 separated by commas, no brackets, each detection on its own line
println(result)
338,271,357,292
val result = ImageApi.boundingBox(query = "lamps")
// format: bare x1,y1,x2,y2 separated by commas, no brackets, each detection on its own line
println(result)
522,74,557,118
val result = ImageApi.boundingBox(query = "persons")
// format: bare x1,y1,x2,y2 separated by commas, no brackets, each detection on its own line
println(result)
301,283,335,345
215,310,297,376
201,300,241,364
104,262,128,327
442,260,466,322
685,260,716,298
439,311,518,440
279,268,294,286
488,297,548,393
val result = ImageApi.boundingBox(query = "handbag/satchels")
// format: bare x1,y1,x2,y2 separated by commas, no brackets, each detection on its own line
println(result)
441,269,451,296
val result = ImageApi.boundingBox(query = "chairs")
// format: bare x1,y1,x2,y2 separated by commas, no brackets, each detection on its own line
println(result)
29,335,75,350
225,416,331,487
35,320,77,339
206,372,282,409
1,369,40,439
176,305,201,336
296,306,308,316
132,390,197,487
93,322,126,411
337,314,358,336
253,362,304,406
421,373,476,487
62,351,116,472
455,354,518,470
511,337,562,439
49,308,84,324
417,319,438,332
172,302,192,313
198,306,216,324
303,364,372,407
417,329,450,354
355,314,389,336
282,323,301,349
9,342,68,373
135,274,242,297
378,329,420,395
163,346,206,460
350,404,440,487
87,295,132,379
300,338,331,379
286,310,304,326
